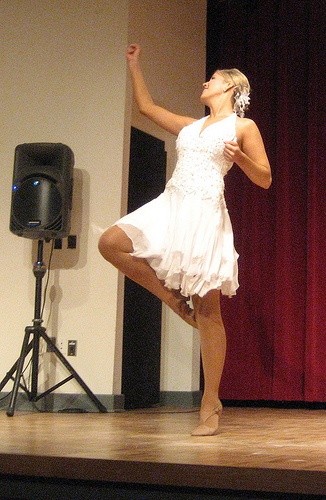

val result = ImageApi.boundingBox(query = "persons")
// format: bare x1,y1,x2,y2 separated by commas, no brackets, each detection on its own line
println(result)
97,43,271,436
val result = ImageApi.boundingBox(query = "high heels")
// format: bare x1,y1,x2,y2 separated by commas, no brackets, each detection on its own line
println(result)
169,289,198,329
191,403,222,436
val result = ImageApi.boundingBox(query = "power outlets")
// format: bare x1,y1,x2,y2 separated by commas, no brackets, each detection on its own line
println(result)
47,337,55,352
68,340,76,356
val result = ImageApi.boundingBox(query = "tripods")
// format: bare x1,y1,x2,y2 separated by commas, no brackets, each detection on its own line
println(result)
0,243,107,417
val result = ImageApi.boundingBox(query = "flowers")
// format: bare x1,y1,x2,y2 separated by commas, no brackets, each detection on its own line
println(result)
233,91,251,119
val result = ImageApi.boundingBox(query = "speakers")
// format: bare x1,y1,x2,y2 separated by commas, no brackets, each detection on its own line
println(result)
9,142,75,243
